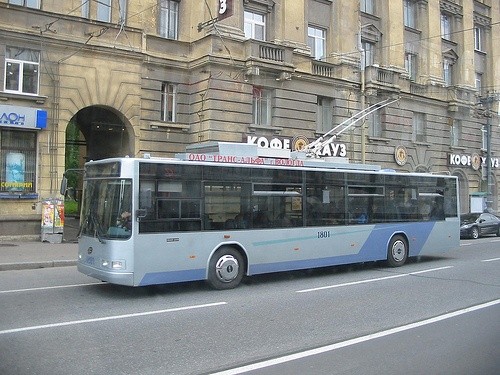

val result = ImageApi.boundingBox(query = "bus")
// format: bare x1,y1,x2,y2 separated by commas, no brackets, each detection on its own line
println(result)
77,140,461,291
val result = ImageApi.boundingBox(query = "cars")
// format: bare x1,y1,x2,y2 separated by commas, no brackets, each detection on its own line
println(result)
460,213,500,239
